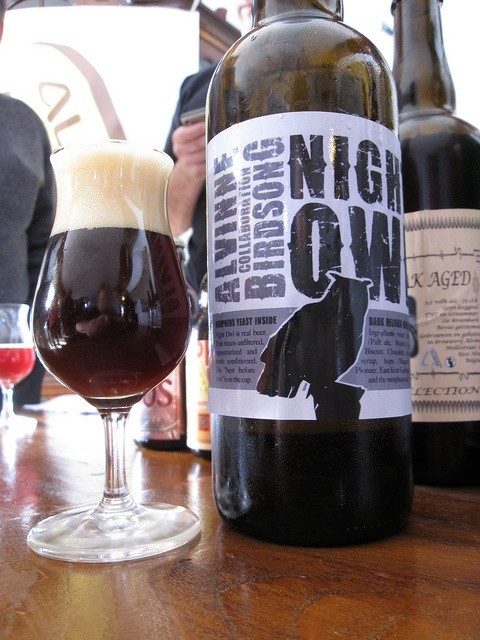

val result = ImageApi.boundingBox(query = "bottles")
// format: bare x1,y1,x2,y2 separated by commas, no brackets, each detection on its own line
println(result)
124,247,196,452
392,2,480,490
183,262,211,458
205,0,411,547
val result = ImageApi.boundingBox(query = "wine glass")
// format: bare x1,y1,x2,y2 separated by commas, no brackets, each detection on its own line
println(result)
1,301,30,434
23,142,199,563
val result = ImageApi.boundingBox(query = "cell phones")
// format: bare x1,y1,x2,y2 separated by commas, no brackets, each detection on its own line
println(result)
179,106,209,127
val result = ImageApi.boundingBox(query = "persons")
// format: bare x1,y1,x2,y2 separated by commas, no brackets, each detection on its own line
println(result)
0,0,56,410
163,66,216,342
74,284,129,339
49,289,74,347
